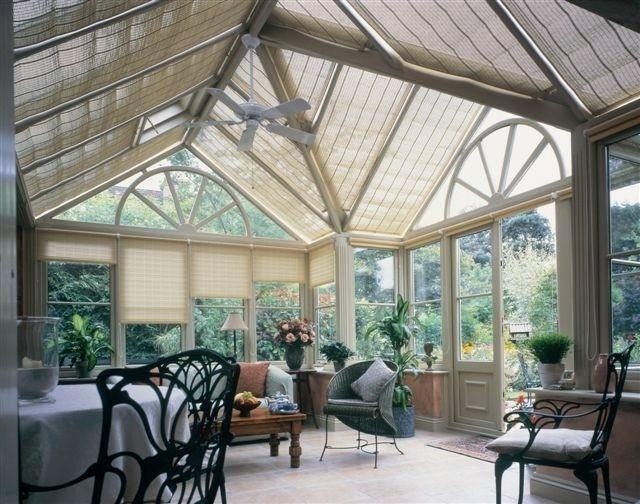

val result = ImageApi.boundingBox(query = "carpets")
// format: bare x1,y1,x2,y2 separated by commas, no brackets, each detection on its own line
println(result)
425,435,498,464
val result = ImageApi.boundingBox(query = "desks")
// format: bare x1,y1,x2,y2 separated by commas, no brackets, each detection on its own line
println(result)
232,410,307,468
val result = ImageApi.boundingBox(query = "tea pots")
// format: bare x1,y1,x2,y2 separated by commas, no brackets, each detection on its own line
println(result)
265,391,289,414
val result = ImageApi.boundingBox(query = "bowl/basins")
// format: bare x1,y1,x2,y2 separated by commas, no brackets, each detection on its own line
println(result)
313,363,324,372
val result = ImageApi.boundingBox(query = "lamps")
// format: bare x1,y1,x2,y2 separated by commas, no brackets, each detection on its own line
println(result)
222,312,247,360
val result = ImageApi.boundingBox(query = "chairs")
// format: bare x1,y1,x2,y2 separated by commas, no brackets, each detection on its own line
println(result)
17,349,243,504
319,359,404,469
486,341,637,504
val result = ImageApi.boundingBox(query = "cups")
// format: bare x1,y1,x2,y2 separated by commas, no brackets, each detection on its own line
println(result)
17,316,62,407
283,403,298,410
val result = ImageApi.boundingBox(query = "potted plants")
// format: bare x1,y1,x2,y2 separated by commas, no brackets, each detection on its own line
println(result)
43,315,115,377
319,342,354,373
365,294,414,438
523,334,573,391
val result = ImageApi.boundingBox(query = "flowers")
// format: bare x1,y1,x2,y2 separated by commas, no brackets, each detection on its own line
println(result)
271,315,315,346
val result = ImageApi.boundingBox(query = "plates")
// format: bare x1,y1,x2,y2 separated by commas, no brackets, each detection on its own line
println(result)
280,409,300,413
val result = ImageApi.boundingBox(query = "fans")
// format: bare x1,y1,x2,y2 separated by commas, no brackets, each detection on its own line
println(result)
181,34,316,153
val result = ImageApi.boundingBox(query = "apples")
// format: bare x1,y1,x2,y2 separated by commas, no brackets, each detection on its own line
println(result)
232,391,259,406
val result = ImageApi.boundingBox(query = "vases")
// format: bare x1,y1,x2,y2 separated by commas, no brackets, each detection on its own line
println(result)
592,354,615,394
286,344,303,370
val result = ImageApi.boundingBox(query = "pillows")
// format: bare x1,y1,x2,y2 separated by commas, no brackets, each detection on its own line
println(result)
351,358,395,401
161,362,294,408
